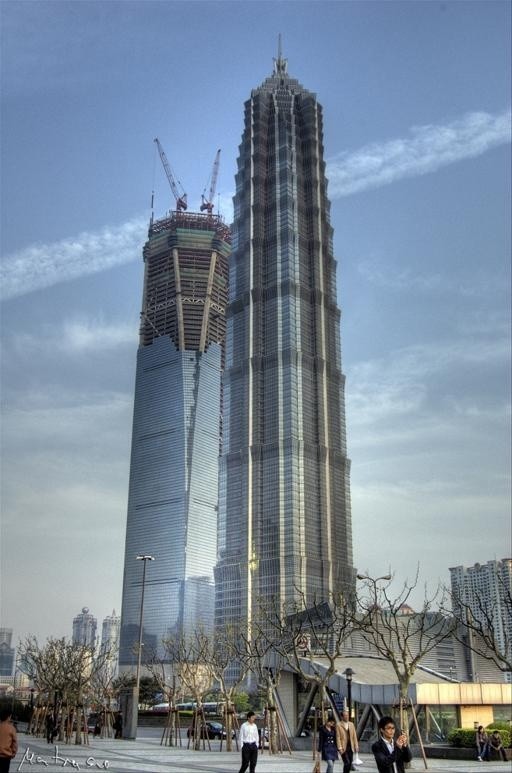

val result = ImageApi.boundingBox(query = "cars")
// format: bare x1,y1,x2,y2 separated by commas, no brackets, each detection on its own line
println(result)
186,719,236,740
259,725,311,744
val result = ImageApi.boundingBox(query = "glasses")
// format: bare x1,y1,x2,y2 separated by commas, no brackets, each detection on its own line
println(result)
384,726,396,729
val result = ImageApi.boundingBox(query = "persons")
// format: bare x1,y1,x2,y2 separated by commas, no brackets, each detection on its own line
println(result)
371,715,413,772
45,708,56,742
113,710,123,738
474,725,489,761
336,710,360,772
236,710,261,772
488,730,510,761
0,707,19,772
92,710,105,738
317,716,339,772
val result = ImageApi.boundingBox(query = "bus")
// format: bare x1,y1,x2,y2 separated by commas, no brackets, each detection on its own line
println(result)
149,700,238,716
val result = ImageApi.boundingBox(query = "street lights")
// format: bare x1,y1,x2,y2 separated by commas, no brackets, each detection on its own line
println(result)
357,572,392,656
135,553,156,687
342,666,356,725
